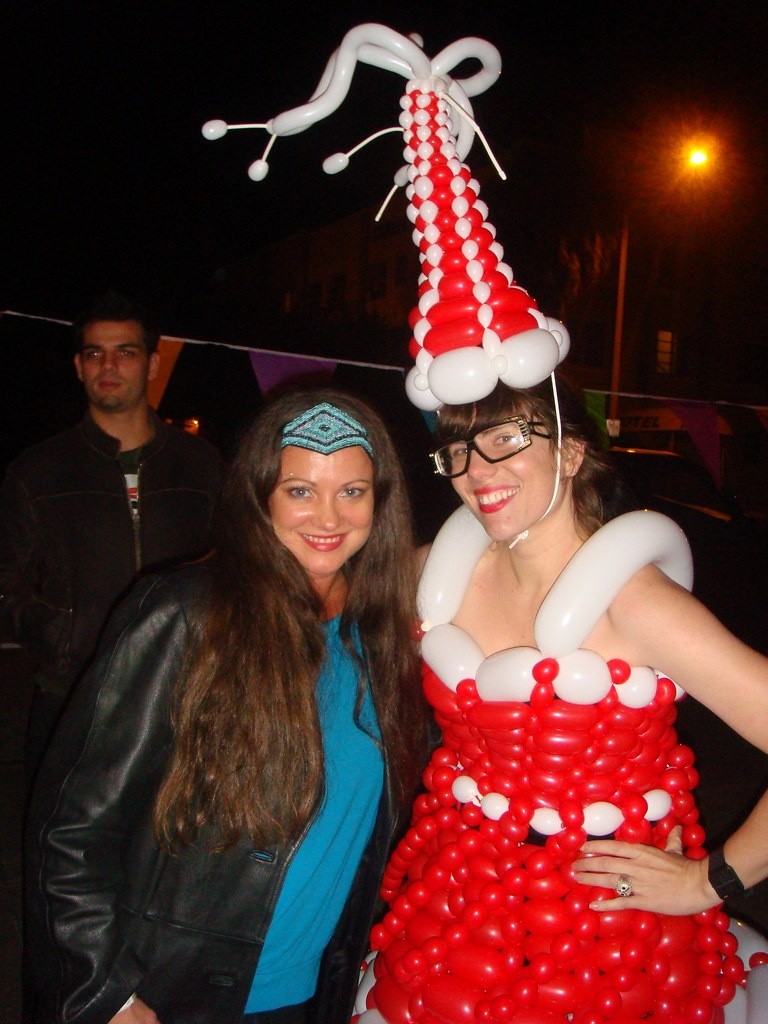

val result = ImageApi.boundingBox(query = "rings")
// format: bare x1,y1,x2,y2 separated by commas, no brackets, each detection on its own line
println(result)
616,875,633,895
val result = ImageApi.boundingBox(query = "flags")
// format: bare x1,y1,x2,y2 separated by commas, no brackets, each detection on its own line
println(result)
249,350,337,402
585,392,610,456
663,400,720,486
756,409,768,432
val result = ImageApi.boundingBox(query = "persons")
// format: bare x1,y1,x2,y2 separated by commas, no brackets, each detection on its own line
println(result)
0,288,224,782
28,391,427,1024
347,383,768,1024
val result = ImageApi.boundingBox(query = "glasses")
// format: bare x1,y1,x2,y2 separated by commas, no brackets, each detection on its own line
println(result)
436,414,549,478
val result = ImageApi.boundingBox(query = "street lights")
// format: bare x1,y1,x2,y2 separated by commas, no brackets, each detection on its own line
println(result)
609,136,712,423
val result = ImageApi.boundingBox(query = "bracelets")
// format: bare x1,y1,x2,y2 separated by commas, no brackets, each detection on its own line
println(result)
708,849,744,902
